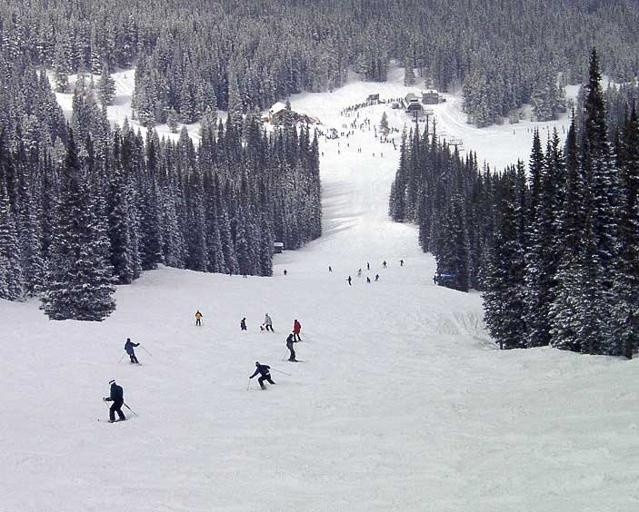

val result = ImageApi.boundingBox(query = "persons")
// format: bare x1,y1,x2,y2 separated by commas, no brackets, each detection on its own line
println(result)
345,275,352,286
292,320,301,341
357,268,362,278
194,310,203,325
366,261,370,270
374,274,379,281
263,313,273,331
284,269,287,275
250,361,275,390
383,261,387,269
241,317,247,330
400,259,403,266
366,276,371,284
124,338,139,363
329,266,332,272
286,334,297,361
104,380,126,423
